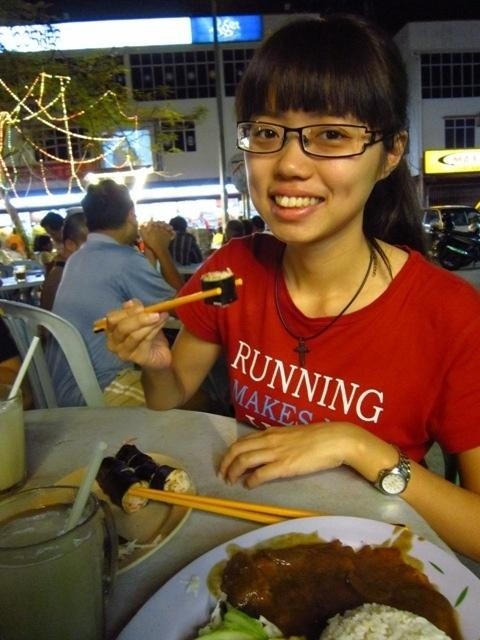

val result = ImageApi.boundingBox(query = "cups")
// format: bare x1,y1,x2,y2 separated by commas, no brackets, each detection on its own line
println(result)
1,486,120,640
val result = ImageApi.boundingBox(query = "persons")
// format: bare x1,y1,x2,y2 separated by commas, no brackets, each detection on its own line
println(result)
41,211,89,348
168,215,203,267
208,214,265,254
0,212,64,267
104,10,480,564
41,179,212,412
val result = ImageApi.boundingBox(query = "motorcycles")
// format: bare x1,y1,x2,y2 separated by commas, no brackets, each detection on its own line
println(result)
436,211,480,272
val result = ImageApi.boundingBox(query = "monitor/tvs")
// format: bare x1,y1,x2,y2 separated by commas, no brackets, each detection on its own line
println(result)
93,119,164,173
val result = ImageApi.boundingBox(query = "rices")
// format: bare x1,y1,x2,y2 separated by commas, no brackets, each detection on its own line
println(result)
321,603,451,639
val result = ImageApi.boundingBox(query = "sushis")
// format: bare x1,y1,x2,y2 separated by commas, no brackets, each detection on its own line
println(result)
100,445,189,514
201,267,235,307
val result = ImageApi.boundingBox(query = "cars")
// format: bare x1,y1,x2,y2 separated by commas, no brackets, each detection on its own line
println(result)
422,204,480,253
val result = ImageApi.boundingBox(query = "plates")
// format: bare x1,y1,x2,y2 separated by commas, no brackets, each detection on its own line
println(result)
113,516,478,640
50,453,197,578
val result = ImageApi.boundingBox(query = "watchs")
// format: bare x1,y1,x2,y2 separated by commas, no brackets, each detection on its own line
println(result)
372,444,411,493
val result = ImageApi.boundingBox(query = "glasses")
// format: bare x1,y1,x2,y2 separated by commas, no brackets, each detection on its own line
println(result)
234,120,393,159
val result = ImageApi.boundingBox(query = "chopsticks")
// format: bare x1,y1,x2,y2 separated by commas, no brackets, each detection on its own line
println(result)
89,278,243,333
128,487,381,526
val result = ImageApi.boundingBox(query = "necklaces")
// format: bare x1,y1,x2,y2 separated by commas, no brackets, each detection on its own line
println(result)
274,244,371,367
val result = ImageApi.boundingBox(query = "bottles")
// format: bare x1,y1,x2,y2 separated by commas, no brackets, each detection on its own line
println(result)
13,264,28,281
1,384,27,499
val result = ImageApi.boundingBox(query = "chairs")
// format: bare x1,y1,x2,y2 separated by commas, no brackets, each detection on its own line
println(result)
0,299,104,409
